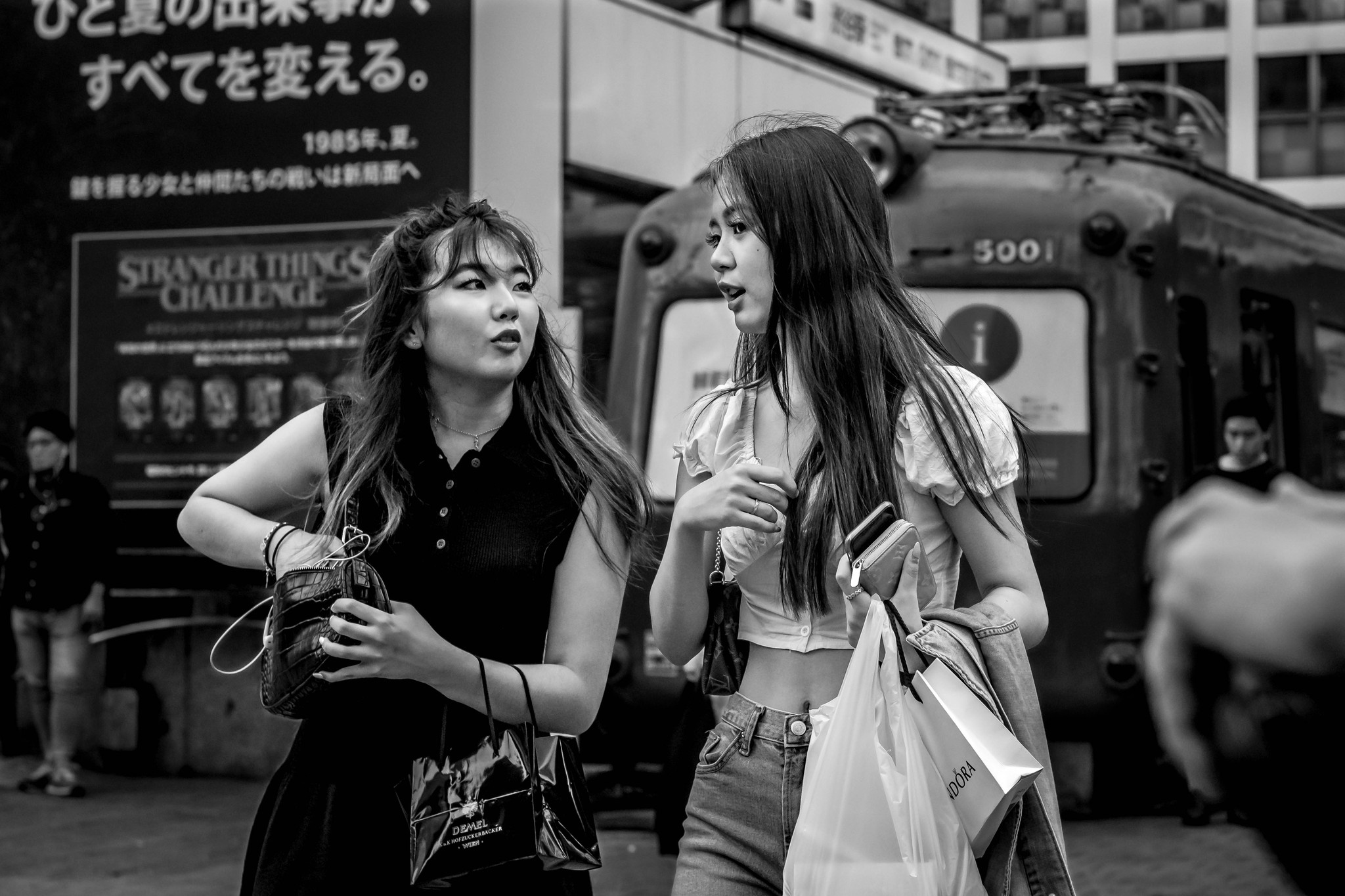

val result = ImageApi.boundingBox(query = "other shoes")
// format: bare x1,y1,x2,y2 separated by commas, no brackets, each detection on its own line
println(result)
19,762,51,791
47,764,84,797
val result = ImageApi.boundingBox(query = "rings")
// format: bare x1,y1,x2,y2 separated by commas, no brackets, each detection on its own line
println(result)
846,587,864,600
751,499,759,515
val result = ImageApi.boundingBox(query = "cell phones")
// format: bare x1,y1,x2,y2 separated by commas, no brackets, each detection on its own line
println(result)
845,501,899,565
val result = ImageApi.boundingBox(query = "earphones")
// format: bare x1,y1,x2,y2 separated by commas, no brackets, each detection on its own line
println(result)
262,618,273,650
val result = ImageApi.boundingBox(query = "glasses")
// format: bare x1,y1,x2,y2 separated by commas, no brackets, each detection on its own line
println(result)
29,439,49,448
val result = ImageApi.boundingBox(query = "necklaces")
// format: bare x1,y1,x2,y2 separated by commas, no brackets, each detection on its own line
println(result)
428,407,503,452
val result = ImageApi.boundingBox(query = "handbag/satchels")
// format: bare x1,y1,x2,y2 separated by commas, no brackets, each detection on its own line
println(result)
408,657,602,885
782,594,989,896
884,595,1043,859
697,458,748,694
253,395,392,716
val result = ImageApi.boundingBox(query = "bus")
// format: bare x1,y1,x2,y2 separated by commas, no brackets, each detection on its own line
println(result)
607,78,1345,857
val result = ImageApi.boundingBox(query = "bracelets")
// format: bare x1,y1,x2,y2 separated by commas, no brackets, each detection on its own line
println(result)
260,522,304,588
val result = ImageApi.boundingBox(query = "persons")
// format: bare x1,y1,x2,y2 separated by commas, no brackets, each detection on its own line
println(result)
177,197,655,896
1146,398,1345,832
651,113,1048,896
0,405,115,798
